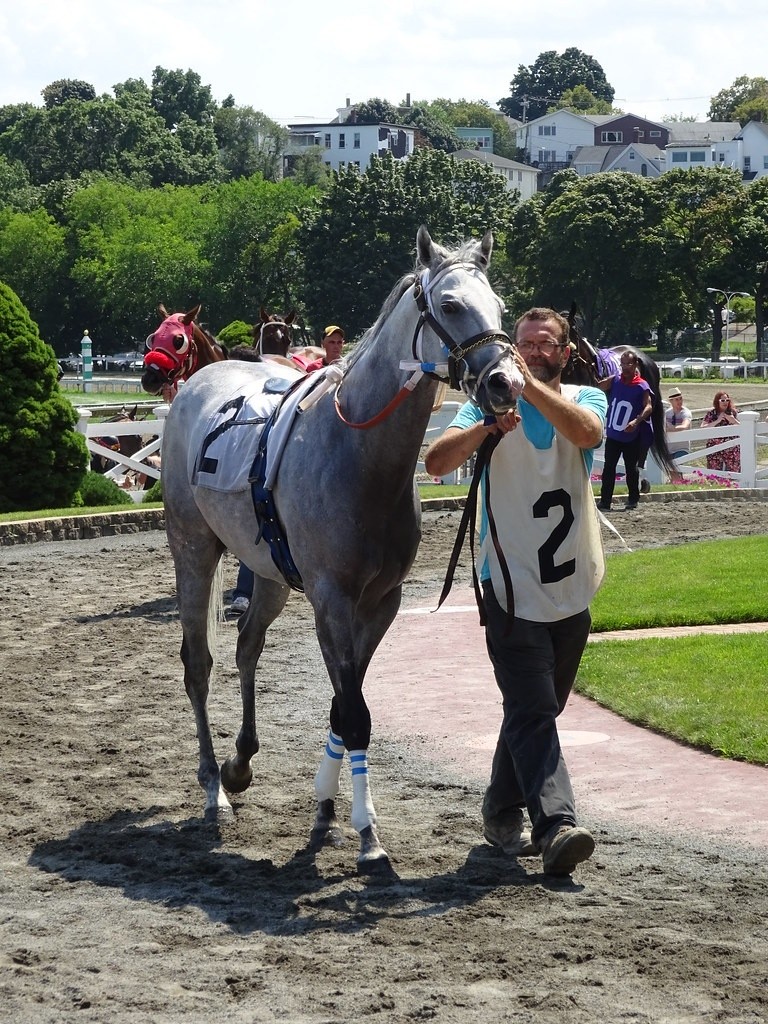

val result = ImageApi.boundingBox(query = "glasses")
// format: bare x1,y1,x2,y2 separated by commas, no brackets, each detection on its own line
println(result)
672,414,676,425
719,399,730,403
517,340,564,354
619,363,635,368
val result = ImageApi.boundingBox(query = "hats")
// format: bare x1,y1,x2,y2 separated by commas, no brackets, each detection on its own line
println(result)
667,387,687,399
322,326,344,340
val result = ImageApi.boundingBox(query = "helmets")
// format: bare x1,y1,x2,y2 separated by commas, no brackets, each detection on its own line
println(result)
98,437,120,452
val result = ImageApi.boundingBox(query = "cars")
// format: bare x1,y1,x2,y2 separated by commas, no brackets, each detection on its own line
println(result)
654,356,768,378
57,351,145,373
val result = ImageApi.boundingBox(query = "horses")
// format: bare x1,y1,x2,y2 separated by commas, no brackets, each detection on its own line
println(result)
139,223,679,878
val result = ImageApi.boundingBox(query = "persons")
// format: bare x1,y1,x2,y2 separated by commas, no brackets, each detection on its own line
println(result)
700,392,741,472
423,306,628,875
663,387,692,479
163,345,263,613
90,436,121,475
306,326,344,373
592,352,655,510
137,434,161,490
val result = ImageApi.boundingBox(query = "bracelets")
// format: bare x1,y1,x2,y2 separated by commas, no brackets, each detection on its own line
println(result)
636,415,642,421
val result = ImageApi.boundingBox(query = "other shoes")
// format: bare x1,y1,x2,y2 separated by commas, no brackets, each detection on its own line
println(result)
231,596,249,613
626,501,639,509
597,502,610,510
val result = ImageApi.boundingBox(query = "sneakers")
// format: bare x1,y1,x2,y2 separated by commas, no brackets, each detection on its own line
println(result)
540,823,594,875
483,825,539,857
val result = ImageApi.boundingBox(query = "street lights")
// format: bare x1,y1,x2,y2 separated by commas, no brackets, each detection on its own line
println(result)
707,288,750,365
